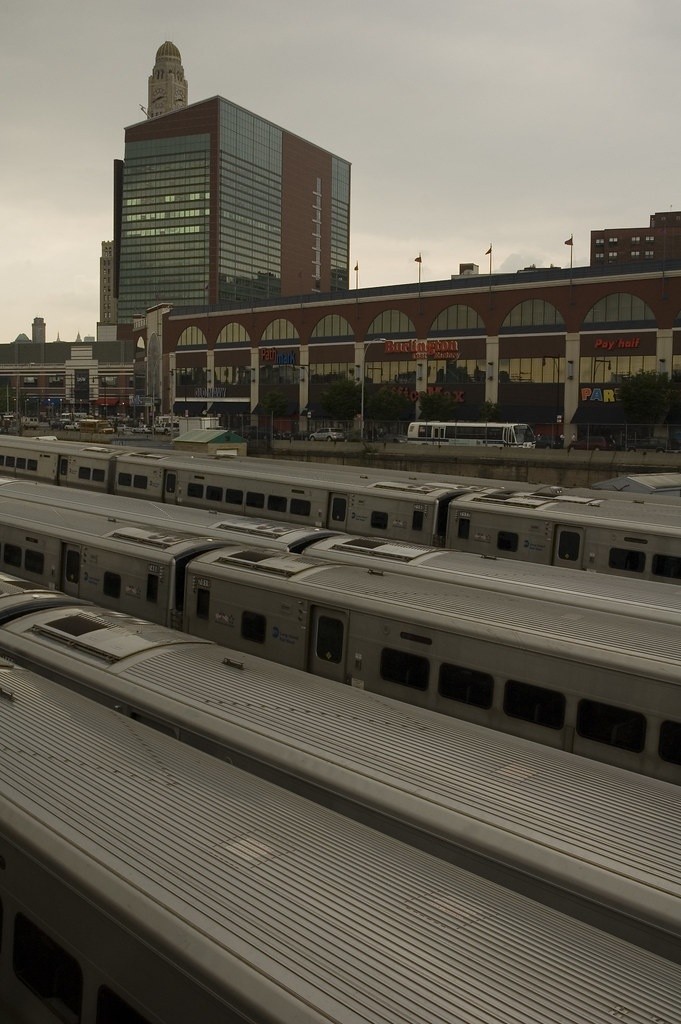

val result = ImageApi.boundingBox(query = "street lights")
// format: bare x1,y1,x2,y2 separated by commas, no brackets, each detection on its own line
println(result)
361,338,386,442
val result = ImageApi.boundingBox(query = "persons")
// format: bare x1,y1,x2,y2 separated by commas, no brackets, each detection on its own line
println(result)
572,433,576,447
560,433,565,449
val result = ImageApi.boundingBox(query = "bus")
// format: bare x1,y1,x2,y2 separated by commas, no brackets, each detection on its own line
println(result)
409,422,535,448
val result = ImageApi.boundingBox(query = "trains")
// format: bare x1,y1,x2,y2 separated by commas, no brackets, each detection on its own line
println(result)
0,434,681,1023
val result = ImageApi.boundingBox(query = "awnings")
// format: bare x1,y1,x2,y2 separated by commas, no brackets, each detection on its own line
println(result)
42,399,63,406
399,407,415,421
169,402,206,414
453,405,481,420
96,399,119,406
569,407,630,424
501,406,564,424
301,402,329,416
207,402,249,414
252,401,298,417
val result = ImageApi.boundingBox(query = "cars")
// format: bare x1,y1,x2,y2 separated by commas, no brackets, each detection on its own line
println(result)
117,422,179,435
309,428,345,442
63,422,81,431
